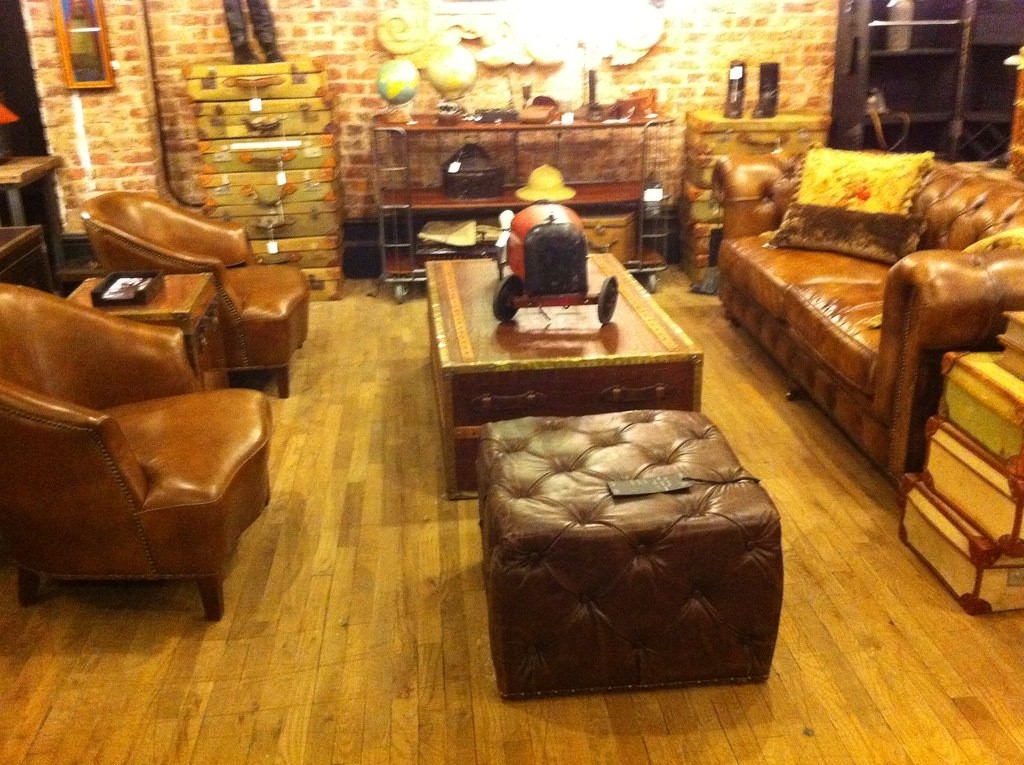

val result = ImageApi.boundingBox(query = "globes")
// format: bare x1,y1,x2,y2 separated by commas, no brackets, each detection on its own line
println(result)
425,44,480,119
376,59,421,124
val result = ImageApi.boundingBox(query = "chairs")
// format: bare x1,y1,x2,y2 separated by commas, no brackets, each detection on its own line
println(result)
78,192,310,397
0,282,275,624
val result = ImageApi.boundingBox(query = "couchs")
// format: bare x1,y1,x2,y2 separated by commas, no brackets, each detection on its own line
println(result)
711,141,1024,488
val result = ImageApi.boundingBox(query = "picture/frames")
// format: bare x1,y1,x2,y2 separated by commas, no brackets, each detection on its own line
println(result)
50,0,116,89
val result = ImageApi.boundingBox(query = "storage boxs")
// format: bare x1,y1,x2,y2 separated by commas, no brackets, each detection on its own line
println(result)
938,350,1024,478
91,270,166,307
579,210,636,260
898,473,1024,616
920,416,1024,558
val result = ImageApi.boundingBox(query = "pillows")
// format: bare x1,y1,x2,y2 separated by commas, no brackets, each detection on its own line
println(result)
771,149,935,263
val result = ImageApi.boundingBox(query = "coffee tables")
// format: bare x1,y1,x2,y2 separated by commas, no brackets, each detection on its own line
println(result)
424,251,705,500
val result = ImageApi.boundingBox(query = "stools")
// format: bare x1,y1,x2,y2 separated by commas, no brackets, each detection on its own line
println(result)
473,409,784,702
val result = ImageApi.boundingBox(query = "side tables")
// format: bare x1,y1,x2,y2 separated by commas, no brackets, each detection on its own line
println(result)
67,271,228,391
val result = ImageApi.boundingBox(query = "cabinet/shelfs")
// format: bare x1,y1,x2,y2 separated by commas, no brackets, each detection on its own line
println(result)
368,106,670,304
827,0,1024,162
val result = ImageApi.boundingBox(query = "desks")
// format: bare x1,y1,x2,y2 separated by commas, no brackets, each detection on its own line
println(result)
0,155,67,289
0,225,55,293
